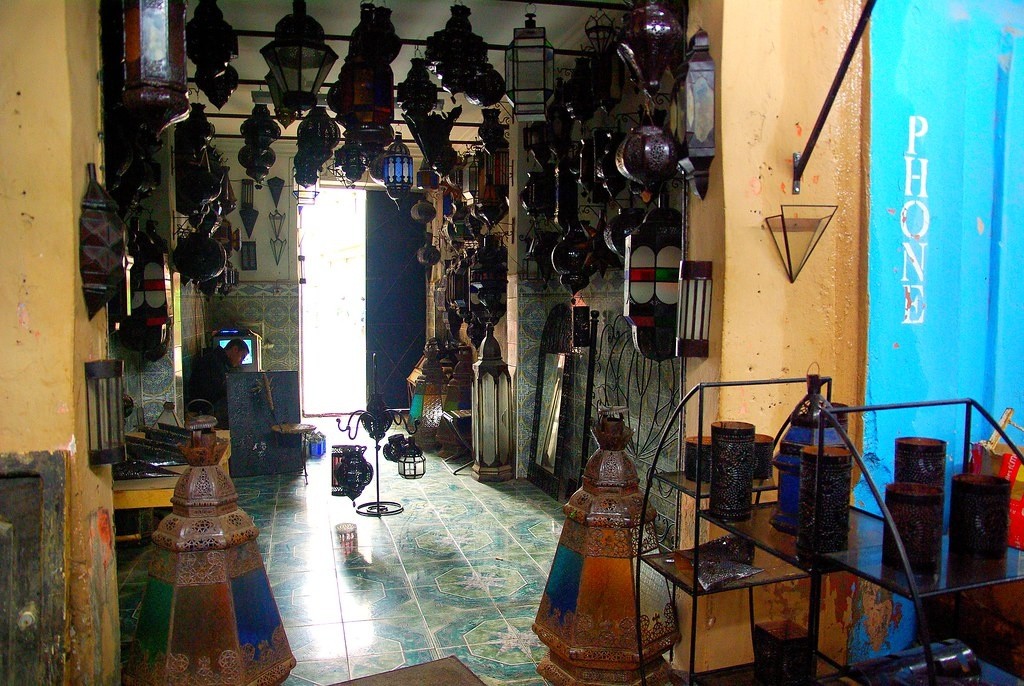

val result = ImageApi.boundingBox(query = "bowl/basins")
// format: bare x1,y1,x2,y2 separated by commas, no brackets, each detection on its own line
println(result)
684,435,775,483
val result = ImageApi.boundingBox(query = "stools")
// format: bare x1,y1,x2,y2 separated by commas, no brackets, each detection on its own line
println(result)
442,409,475,475
271,424,318,484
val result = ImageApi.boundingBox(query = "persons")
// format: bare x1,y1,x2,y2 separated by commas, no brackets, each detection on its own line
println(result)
190,338,249,432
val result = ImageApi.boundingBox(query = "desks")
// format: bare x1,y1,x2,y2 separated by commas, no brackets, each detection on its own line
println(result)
114,431,233,510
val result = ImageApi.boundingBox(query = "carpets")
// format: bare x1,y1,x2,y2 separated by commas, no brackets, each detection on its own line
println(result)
328,653,487,686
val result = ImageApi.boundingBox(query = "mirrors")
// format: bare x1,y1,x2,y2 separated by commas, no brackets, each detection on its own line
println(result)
527,301,571,501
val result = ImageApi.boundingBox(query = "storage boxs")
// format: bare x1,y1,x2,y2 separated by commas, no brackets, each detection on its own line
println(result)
947,438,1024,629
309,440,326,456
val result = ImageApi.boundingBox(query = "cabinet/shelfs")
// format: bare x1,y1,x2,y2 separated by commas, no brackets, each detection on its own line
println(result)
636,377,1024,686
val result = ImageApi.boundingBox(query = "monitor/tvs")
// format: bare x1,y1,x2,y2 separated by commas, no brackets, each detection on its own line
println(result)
213,337,261,373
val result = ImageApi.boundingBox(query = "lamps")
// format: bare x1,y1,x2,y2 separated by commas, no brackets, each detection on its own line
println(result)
80,0,716,686
770,359,846,536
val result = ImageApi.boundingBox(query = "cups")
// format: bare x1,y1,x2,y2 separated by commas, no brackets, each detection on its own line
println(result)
953,475,1010,559
798,447,853,551
710,421,755,520
882,483,944,574
895,436,946,489
